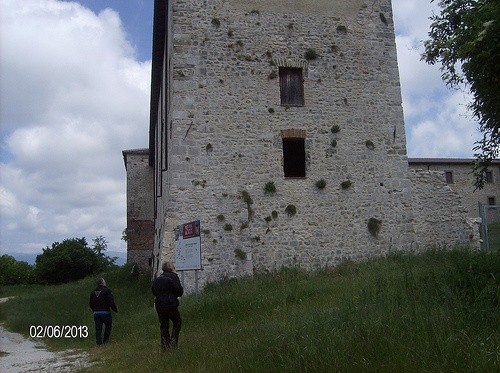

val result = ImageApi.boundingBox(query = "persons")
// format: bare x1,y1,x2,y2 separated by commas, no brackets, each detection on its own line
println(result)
88,277,119,346
152,261,185,353
